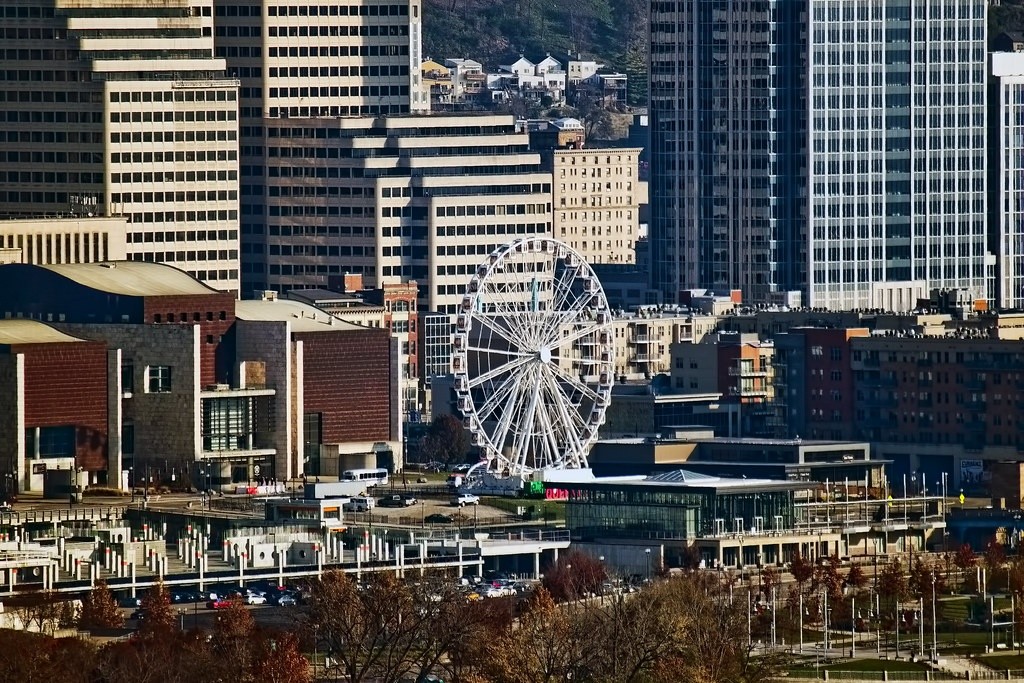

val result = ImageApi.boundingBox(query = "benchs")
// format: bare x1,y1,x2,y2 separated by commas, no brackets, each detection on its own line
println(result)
924,659,939,666
997,643,1009,651
1014,643,1021,650
894,656,906,661
784,648,795,654
879,655,890,660
815,644,824,648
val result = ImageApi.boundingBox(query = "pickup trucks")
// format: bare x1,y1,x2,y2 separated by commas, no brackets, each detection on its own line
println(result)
450,494,480,507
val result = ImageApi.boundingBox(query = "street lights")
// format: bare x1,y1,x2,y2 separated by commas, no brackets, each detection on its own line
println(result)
598,555,604,605
644,547,651,587
565,564,572,615
756,553,762,601
130,466,134,501
200,490,205,517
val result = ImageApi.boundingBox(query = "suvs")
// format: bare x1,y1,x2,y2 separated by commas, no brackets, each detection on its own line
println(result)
378,494,401,507
343,497,376,513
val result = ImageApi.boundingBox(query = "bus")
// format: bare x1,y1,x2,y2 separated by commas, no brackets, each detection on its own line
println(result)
496,578,541,589
340,467,389,487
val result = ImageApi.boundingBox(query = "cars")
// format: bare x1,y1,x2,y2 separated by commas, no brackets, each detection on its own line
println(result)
0,506,20,517
402,496,418,507
425,461,446,473
622,583,637,593
602,582,618,595
420,574,531,603
451,463,471,473
120,584,299,610
